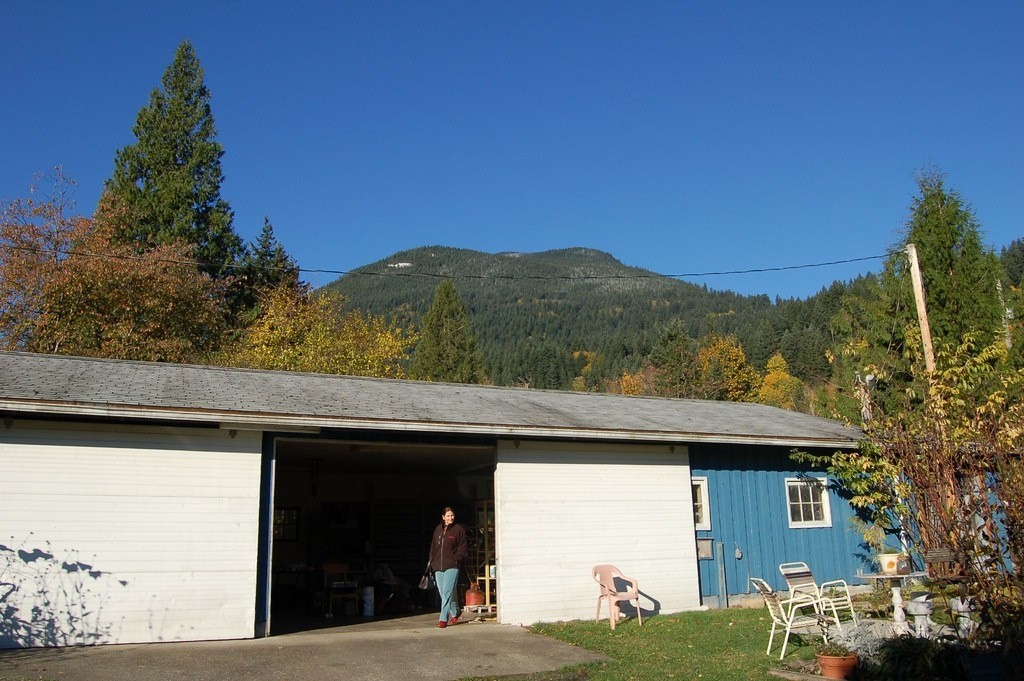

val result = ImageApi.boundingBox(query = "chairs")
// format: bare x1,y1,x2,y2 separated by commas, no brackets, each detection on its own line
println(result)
779,560,859,632
592,564,642,631
750,577,844,662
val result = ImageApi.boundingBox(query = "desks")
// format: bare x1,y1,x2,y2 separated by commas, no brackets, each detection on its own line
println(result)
853,573,928,622
276,565,348,627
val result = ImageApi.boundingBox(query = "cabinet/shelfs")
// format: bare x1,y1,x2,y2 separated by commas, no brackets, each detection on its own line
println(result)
474,500,496,605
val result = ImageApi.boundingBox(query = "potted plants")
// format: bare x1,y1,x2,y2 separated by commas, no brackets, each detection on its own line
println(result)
814,639,859,680
878,548,900,576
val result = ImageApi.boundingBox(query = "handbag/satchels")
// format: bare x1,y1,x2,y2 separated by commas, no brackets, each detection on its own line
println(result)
418,561,437,590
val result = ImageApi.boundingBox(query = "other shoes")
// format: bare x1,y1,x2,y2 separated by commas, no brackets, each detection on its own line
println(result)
437,621,448,628
448,610,462,626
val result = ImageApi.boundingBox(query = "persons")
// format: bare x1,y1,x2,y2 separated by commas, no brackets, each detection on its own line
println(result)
427,507,467,628
